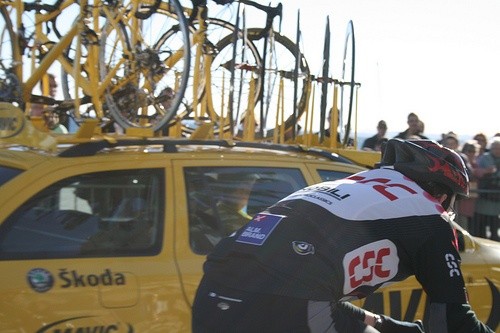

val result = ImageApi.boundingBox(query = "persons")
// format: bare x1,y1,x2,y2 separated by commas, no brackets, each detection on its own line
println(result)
190,138,494,332
361,112,500,244
8,108,322,258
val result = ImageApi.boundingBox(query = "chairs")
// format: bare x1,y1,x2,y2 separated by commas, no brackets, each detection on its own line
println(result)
246,178,295,216
186,174,220,254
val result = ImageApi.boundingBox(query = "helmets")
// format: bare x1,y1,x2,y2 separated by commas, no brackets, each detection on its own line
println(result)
377,137,470,197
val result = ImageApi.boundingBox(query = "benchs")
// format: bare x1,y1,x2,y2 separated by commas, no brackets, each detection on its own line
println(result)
17,205,100,239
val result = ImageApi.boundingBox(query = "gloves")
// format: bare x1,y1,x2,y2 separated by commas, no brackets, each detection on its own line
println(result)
379,314,425,333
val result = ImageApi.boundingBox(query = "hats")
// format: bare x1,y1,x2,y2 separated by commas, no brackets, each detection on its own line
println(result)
157,87,176,101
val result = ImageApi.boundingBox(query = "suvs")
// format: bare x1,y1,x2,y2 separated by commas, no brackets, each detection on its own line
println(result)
0,137,500,333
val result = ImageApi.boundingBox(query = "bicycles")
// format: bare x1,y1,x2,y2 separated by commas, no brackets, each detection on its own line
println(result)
0,0,313,154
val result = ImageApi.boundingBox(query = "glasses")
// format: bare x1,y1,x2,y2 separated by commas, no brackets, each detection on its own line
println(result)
444,202,455,216
